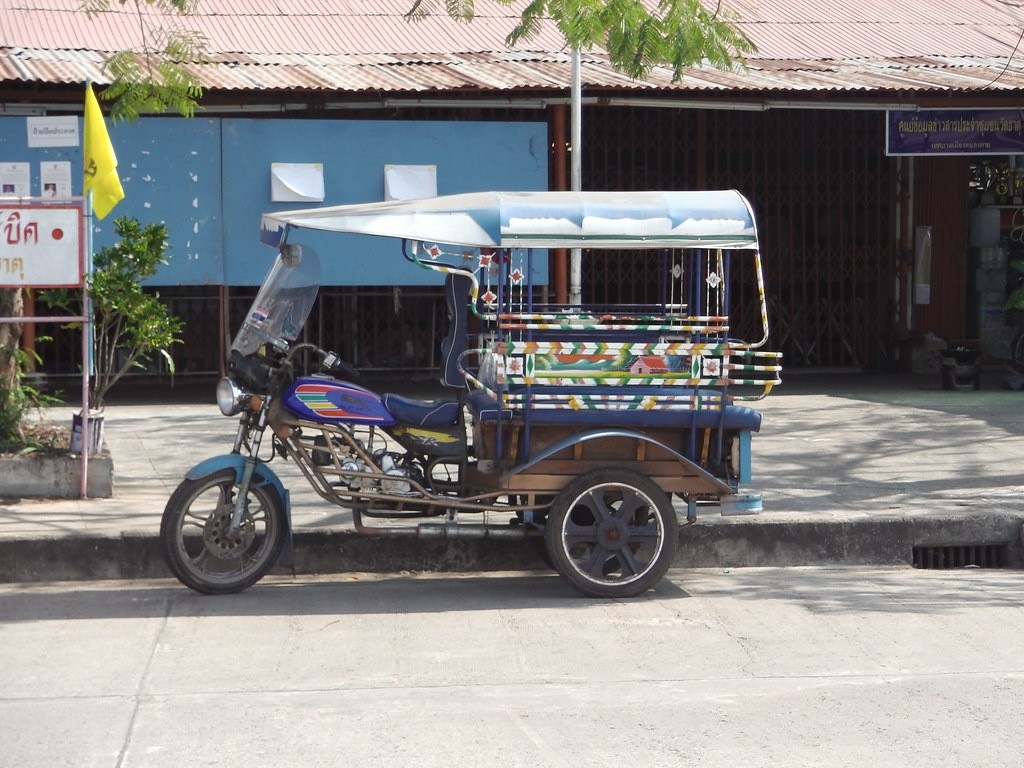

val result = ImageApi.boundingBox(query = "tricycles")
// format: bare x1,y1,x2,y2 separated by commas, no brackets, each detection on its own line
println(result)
158,189,786,600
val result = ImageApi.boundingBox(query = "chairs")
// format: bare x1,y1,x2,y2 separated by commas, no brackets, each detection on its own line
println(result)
384,377,512,455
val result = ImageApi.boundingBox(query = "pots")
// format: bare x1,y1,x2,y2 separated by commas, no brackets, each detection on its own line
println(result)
939,347,981,366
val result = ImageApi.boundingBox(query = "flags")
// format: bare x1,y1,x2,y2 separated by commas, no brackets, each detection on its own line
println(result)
80,85,126,222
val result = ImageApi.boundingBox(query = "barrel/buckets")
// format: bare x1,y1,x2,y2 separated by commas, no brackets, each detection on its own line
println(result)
70,408,104,455
